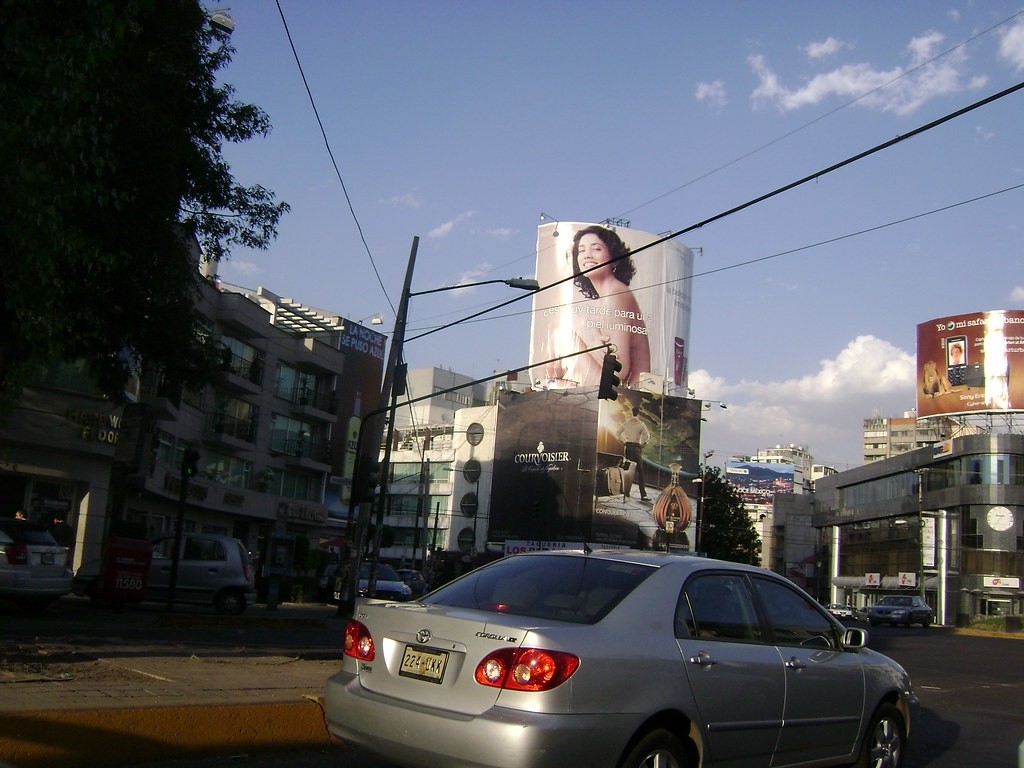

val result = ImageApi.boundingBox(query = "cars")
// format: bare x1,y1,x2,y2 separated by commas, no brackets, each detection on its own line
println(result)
73,533,257,603
0,516,75,603
825,602,852,621
860,595,932,629
396,568,430,597
316,549,921,768
326,558,411,609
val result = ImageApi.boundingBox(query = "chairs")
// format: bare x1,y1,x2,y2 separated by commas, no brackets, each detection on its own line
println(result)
687,583,756,646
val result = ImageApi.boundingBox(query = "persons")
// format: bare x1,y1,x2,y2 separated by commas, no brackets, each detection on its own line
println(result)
14,509,28,520
53,516,75,544
950,344,963,364
847,603,851,607
616,408,652,501
549,225,650,388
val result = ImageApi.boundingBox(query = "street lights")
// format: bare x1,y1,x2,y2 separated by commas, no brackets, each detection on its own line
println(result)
334,277,542,616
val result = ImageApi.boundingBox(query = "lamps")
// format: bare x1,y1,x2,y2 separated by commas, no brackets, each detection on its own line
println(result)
687,387,696,396
696,247,703,255
665,230,671,238
603,218,610,228
648,377,656,385
370,314,383,325
540,213,546,220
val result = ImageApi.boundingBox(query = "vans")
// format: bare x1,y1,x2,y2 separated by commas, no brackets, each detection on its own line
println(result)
430,550,505,591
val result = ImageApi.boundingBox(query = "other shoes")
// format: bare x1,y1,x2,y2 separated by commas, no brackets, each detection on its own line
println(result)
641,495,653,502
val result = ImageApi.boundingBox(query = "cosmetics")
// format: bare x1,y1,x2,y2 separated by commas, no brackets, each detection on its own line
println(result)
674,337,688,397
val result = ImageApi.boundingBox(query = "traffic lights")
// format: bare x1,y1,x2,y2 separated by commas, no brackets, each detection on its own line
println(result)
597,355,622,404
353,457,381,501
181,451,200,478
